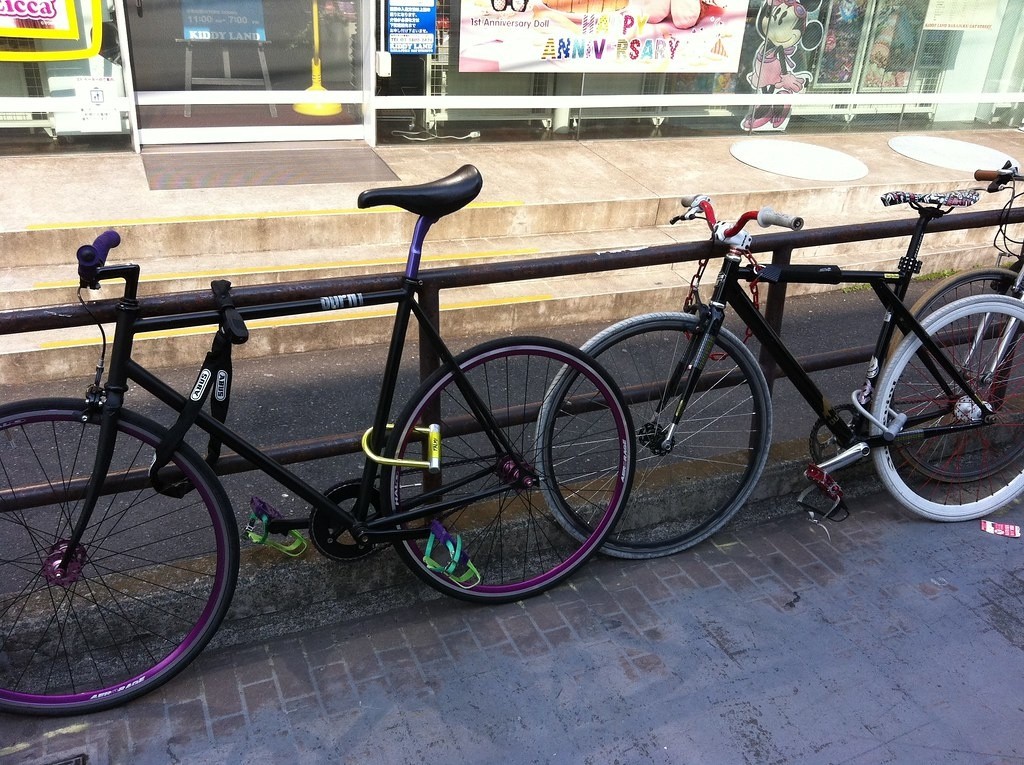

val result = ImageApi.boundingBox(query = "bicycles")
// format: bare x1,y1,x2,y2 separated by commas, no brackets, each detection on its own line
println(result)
0,164,638,716
534,188,1024,560
883,160,1024,483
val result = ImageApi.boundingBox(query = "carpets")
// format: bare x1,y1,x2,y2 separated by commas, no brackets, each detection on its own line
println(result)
140,138,401,191
